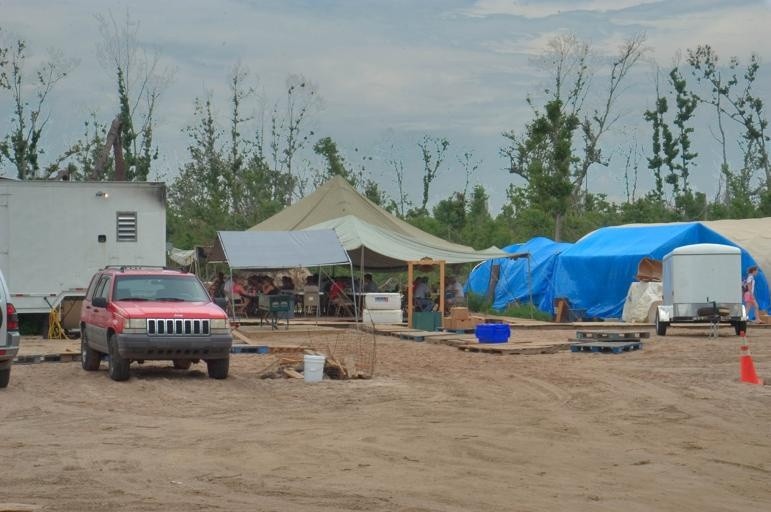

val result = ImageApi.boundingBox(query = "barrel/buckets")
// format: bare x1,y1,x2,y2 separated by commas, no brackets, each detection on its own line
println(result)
304,354,326,383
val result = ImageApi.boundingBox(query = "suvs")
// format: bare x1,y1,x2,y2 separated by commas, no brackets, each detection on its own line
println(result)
80,267,232,382
0,268,21,390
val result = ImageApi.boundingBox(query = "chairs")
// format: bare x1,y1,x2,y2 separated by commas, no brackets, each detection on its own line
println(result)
203,278,472,328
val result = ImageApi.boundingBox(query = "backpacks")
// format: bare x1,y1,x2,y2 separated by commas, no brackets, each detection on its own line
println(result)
741,280,748,292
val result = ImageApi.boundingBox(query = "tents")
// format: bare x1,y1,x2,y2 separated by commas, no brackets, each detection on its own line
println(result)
463,223,771,318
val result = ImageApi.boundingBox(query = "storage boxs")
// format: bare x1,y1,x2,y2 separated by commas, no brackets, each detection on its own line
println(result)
412,312,443,332
472,316,484,328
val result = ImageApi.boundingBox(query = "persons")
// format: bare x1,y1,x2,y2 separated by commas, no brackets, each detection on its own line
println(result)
208,272,461,321
742,262,766,326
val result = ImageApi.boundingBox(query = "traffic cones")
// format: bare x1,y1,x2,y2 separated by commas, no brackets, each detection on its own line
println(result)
738,330,766,387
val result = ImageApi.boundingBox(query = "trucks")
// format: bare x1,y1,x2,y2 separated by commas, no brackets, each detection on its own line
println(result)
0,179,168,338
